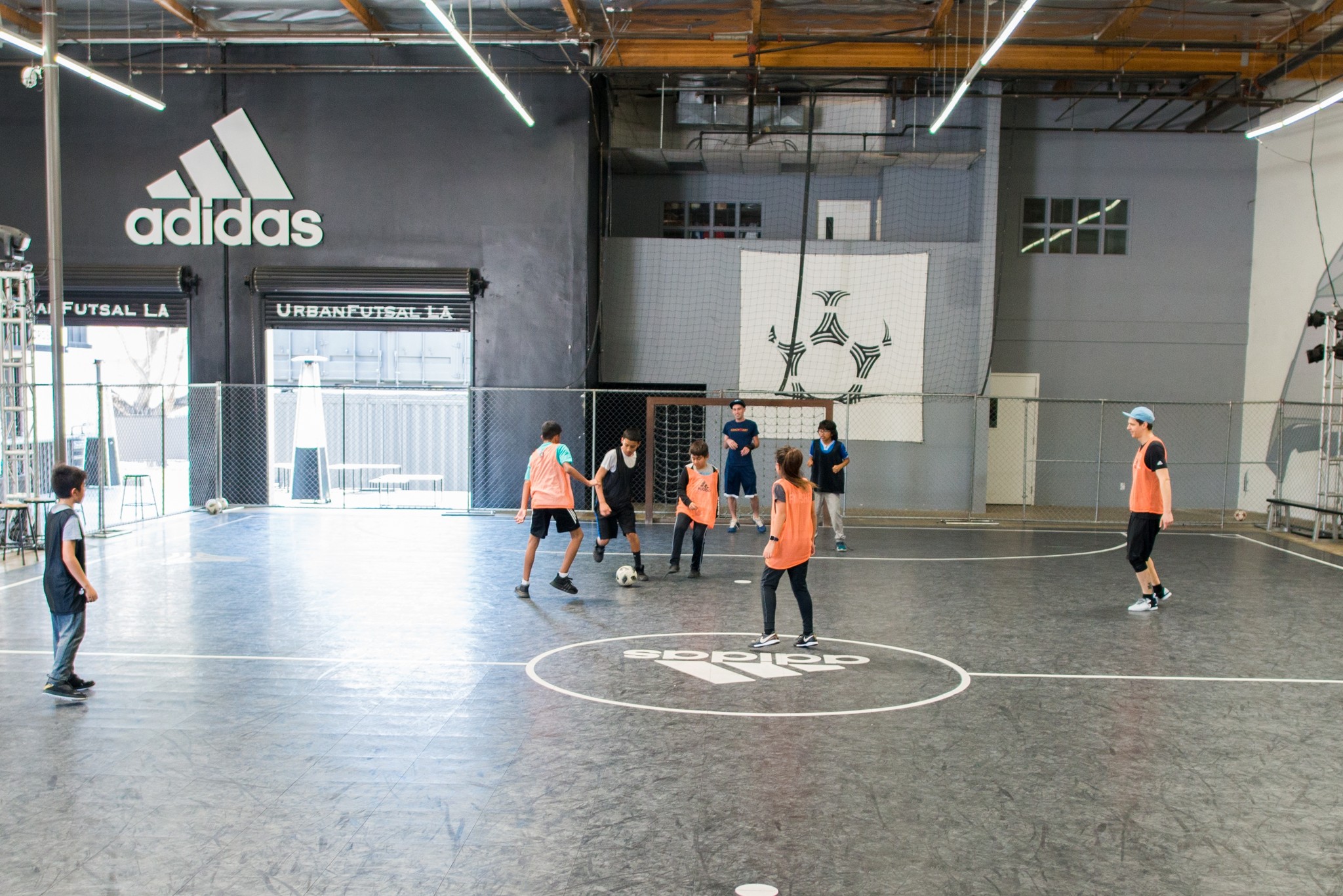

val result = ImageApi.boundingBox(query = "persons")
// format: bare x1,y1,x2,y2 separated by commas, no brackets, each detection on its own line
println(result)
1122,406,1174,611
722,399,767,533
593,429,650,581
807,419,850,552
749,446,821,647
667,440,720,578
43,465,99,700
514,421,602,598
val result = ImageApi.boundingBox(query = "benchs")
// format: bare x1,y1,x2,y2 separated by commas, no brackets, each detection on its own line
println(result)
1267,499,1343,543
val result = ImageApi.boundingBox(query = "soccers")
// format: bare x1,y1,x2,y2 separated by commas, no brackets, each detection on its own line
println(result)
216,498,228,510
207,500,222,515
616,565,638,586
206,499,215,508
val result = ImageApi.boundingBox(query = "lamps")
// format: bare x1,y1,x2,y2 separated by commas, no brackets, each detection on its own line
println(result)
1307,310,1343,364
929,0,1037,135
1246,11,1343,139
0,0,166,111
421,0,535,127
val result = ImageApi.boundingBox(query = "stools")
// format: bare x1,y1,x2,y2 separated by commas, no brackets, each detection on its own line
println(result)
121,475,158,519
0,498,56,566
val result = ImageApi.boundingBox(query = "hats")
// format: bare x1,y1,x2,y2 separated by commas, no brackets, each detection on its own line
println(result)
1123,406,1155,424
729,399,745,409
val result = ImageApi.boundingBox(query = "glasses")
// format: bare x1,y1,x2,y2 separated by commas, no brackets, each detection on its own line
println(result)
818,430,830,434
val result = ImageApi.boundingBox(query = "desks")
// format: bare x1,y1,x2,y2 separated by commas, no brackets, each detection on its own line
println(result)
372,474,444,506
277,463,293,493
328,464,401,494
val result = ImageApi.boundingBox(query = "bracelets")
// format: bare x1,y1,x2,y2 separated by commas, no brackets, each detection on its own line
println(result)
769,535,779,541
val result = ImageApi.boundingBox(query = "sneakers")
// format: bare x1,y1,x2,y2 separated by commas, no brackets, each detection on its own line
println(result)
793,632,818,647
593,545,605,563
66,673,95,690
727,518,740,533
687,571,700,578
515,584,530,597
549,572,578,594
635,565,648,581
668,565,680,573
42,680,89,701
1128,594,1158,611
747,631,780,648
835,541,853,551
753,514,766,533
1156,586,1172,600
